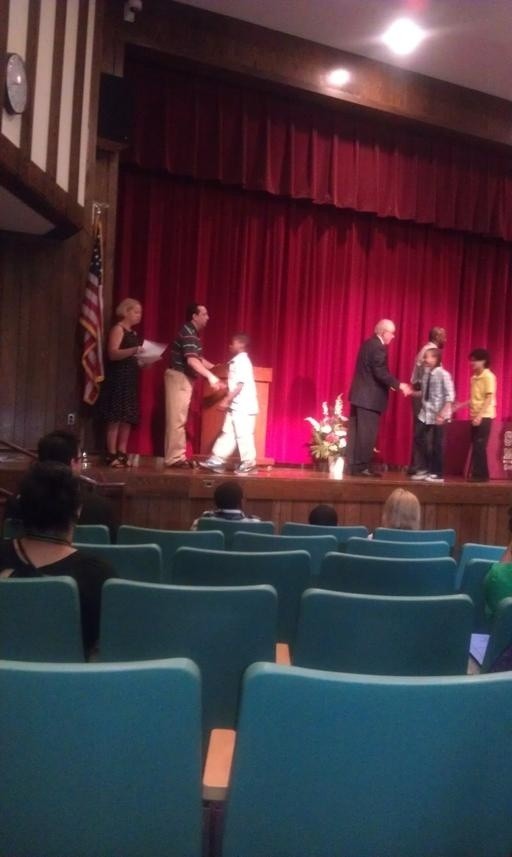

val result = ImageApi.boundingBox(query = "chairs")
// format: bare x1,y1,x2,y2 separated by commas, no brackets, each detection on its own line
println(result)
1,657,216,854
216,662,510,854
1,516,509,726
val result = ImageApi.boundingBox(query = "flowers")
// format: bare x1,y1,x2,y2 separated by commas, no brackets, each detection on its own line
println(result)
302,392,351,462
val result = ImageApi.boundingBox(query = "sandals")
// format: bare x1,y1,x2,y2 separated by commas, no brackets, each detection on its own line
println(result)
104,449,123,468
116,451,133,467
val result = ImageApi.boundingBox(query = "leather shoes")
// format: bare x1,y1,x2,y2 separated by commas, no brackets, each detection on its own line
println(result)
170,459,197,468
353,467,381,477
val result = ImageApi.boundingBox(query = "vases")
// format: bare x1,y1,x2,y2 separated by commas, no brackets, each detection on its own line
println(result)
328,455,346,482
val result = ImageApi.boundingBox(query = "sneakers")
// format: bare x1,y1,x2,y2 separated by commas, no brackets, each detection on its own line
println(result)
425,474,444,482
233,462,257,475
199,461,226,474
411,470,430,480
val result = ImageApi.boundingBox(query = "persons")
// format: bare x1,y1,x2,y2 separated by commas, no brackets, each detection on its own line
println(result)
198,331,260,478
94,297,146,469
482,506,512,623
410,347,457,485
366,486,421,540
407,324,449,477
5,427,118,545
348,317,411,476
189,481,262,533
162,301,227,470
0,460,105,661
465,346,498,481
308,505,341,527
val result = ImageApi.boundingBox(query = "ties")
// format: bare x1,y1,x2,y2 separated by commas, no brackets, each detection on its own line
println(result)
425,373,431,401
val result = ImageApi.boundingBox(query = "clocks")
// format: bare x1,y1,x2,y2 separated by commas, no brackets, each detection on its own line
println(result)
2,51,31,117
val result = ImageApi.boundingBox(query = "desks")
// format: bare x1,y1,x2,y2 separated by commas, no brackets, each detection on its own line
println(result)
443,415,511,480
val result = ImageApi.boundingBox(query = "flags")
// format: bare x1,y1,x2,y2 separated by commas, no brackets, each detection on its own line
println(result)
72,220,104,418
